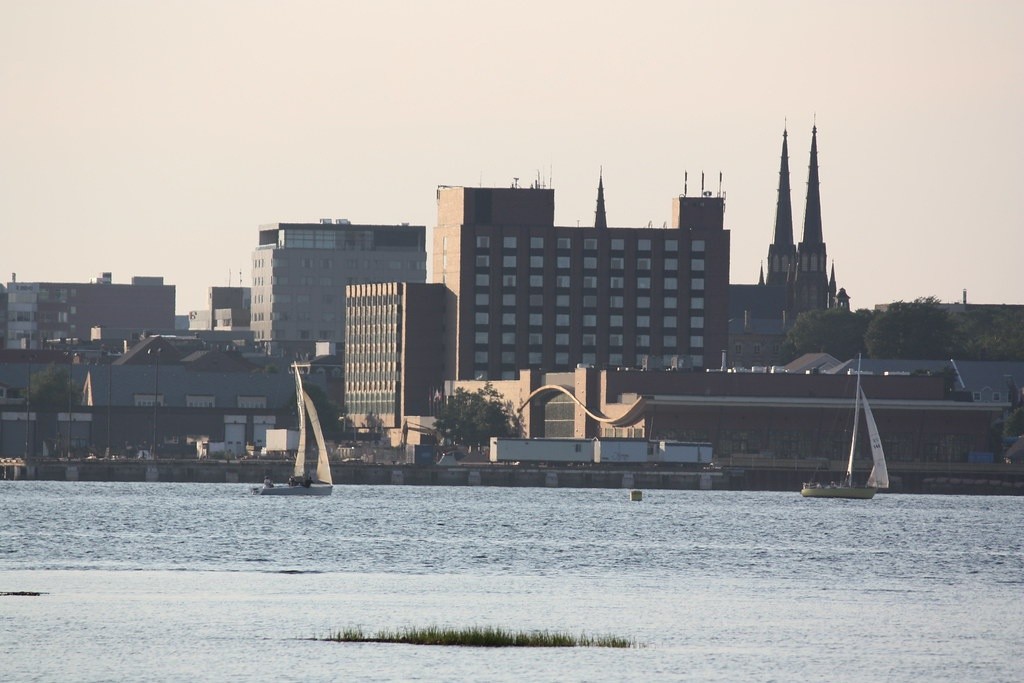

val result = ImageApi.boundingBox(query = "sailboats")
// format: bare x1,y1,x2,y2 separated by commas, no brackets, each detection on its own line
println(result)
800,352,890,499
250,358,334,496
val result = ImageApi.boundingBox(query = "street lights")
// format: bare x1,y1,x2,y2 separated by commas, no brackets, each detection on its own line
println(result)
146,347,163,463
23,354,38,462
63,350,81,464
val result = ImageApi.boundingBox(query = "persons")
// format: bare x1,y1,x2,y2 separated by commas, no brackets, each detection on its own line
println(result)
846,472,850,485
288,476,313,488
264,477,274,488
